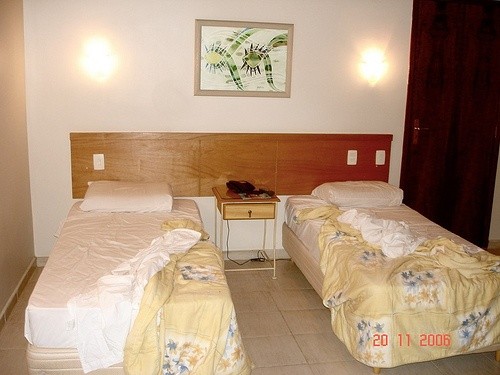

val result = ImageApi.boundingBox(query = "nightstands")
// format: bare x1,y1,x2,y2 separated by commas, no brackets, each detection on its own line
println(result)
213,186,281,280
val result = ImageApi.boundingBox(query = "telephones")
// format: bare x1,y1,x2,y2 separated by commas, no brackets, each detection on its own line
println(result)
225,179,255,194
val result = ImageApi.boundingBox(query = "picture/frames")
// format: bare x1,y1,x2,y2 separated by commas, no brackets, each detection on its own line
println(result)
192,19,295,98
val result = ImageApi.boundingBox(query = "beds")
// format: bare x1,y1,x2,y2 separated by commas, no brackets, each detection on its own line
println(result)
24,200,256,375
283,196,500,372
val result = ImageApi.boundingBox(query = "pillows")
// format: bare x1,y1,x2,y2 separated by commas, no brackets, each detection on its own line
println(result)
79,180,174,213
309,180,403,208
